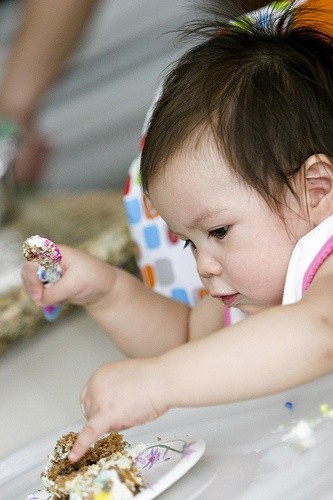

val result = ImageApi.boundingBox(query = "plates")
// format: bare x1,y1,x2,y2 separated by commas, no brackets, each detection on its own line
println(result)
27,438,206,500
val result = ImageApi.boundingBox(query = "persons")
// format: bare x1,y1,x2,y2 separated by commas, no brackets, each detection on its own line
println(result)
20,2,332,466
1,1,264,196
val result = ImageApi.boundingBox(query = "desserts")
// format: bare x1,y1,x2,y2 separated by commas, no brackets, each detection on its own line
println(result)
40,429,144,500
